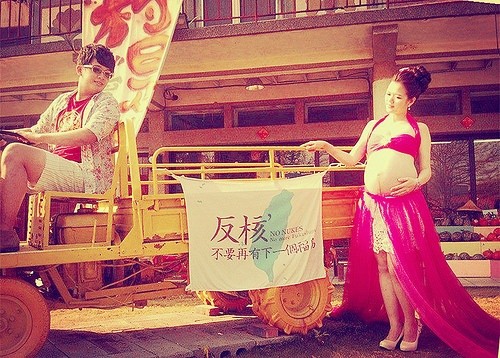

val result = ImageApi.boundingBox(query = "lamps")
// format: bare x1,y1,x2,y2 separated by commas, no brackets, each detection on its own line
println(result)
244,77,266,91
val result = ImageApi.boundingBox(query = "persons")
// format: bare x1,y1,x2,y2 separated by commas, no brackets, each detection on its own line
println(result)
0,44,120,253
300,65,500,355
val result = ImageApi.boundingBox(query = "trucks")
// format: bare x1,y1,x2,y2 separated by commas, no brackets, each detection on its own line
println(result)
0,116,367,358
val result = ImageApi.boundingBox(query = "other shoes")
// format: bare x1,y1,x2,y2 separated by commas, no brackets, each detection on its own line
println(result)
399,318,423,351
380,323,405,350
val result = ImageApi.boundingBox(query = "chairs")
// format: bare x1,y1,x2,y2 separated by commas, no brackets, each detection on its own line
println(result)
27,120,126,249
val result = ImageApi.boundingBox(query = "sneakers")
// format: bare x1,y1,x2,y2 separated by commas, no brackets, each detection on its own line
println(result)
0,229,20,252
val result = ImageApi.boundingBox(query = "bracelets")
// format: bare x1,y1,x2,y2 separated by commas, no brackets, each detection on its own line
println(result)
416,178,420,189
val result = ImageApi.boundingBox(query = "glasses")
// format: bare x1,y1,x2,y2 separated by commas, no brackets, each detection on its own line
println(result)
78,65,113,79
